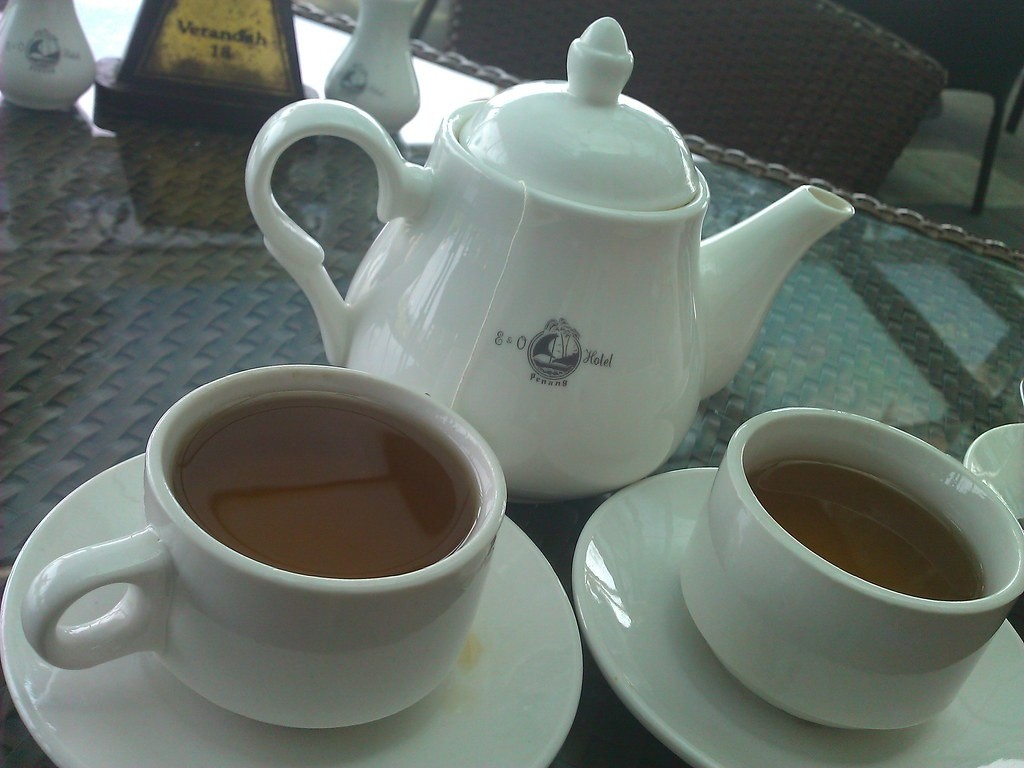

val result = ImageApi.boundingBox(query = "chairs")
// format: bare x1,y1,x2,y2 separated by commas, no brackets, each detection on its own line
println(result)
408,0,948,199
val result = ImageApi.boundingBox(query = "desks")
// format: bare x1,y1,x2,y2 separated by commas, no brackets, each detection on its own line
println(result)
0,0,1024,768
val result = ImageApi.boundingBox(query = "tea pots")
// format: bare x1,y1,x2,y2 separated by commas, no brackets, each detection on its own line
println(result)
244,15,856,498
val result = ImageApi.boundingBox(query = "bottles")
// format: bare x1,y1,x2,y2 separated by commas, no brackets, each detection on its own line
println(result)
0,1,95,112
324,1,422,132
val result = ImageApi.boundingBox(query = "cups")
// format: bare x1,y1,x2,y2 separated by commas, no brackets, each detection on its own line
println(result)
21,364,507,728
678,407,1023,730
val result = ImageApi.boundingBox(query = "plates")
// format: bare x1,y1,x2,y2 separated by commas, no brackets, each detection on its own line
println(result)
963,422,1024,528
573,466,1024,768
0,454,583,768
